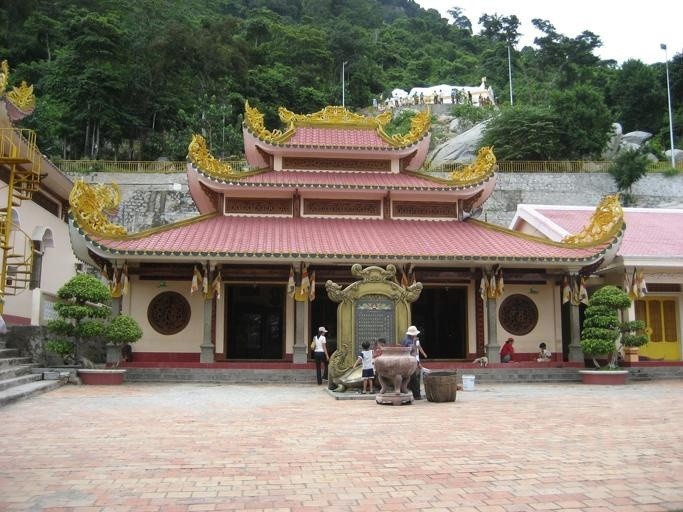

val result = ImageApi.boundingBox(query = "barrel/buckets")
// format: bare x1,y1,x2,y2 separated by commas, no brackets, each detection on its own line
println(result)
462,374,475,391
423,370,457,403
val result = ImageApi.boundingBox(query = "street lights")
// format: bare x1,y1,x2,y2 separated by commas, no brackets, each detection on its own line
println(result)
661,44,674,169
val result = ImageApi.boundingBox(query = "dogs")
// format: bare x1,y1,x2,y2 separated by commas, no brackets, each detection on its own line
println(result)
472,356,488,367
77,355,97,368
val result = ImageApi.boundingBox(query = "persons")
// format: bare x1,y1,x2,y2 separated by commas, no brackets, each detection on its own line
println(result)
353,340,375,394
501,338,515,362
312,327,330,385
399,325,426,400
376,338,386,346
537,342,552,362
384,88,491,109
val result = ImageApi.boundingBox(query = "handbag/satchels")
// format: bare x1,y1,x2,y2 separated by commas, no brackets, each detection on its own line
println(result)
311,342,315,350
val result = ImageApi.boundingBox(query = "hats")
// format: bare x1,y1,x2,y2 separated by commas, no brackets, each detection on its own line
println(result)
319,327,328,333
406,326,420,335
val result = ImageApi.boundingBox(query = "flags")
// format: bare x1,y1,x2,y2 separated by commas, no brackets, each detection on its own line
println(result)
625,268,649,300
479,270,505,303
190,264,222,300
561,276,590,306
100,259,129,298
392,264,416,288
286,262,316,302
